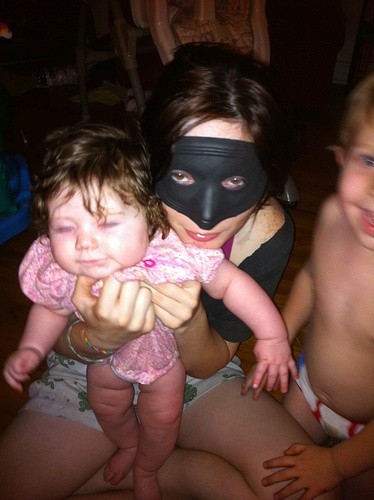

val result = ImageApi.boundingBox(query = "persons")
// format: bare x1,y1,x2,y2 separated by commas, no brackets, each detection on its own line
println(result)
242,75,374,499
2,122,299,500
0,41,294,500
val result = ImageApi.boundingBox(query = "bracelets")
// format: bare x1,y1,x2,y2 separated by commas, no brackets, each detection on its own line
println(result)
66,319,114,363
